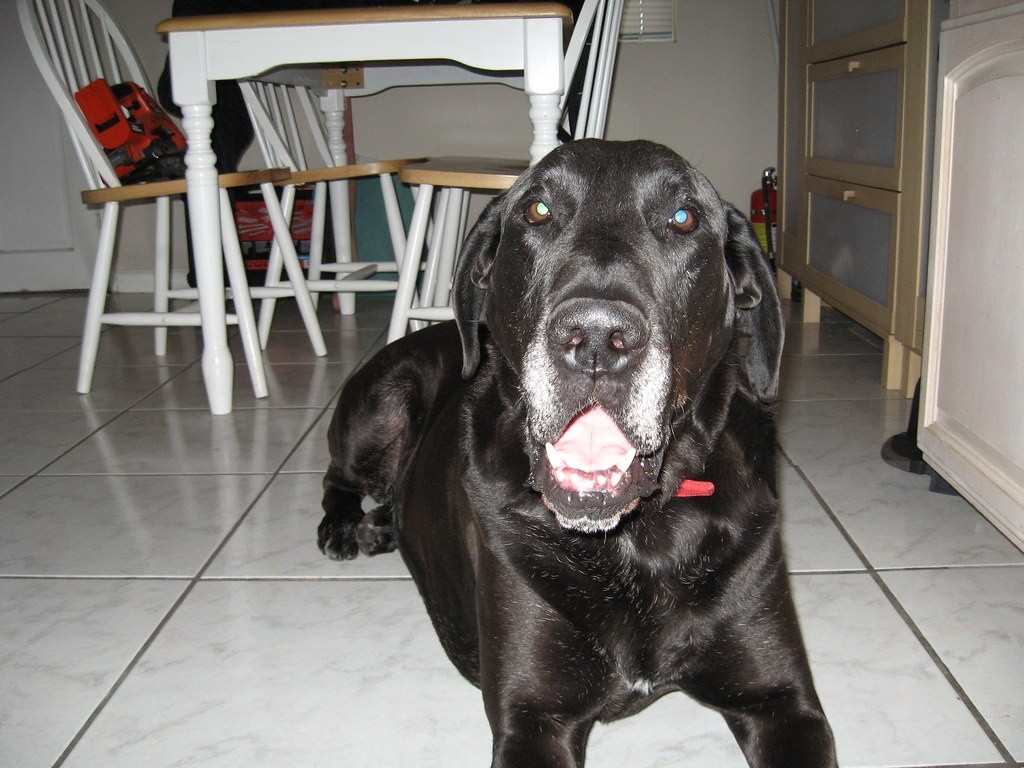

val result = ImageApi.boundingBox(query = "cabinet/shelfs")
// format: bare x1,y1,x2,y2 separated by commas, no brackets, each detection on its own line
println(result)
776,0,1024,550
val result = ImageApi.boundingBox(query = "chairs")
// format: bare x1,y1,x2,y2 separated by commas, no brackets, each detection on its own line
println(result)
389,1,626,344
237,79,434,356
17,1,327,398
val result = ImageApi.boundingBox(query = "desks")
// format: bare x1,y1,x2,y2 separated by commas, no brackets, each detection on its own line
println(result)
157,2,574,416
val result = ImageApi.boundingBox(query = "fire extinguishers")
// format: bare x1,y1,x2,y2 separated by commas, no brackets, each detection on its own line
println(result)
751,168,778,275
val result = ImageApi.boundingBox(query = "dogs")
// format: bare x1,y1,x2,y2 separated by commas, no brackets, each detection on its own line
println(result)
319,138,840,768
